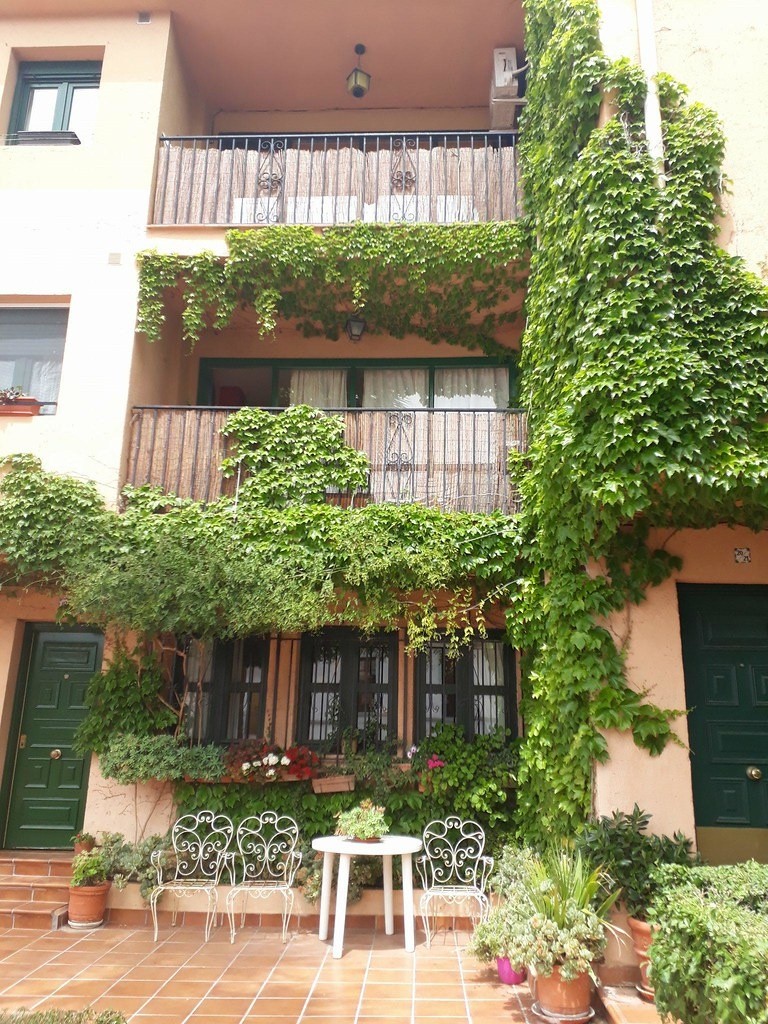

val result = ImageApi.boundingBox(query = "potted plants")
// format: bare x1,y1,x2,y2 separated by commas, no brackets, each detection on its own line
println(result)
575,802,709,993
68,848,111,923
469,844,634,1018
99,732,226,783
312,765,357,794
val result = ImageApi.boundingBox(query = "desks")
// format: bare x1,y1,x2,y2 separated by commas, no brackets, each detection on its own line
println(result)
311,836,424,960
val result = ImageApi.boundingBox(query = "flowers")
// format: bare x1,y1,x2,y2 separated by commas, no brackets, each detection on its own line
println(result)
406,719,524,816
333,798,390,841
69,830,94,846
0,386,24,405
222,738,320,784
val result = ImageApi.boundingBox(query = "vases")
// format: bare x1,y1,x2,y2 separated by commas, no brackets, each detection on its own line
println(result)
0,397,41,417
419,770,519,793
75,841,92,856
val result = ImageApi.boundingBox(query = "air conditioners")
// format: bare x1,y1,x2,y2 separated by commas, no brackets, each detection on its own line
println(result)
489,47,519,131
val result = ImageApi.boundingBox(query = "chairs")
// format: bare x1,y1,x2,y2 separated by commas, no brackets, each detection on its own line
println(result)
151,810,234,943
225,811,302,945
414,816,495,948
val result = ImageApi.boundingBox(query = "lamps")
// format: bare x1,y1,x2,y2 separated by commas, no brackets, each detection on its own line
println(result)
342,314,366,342
346,43,370,96
